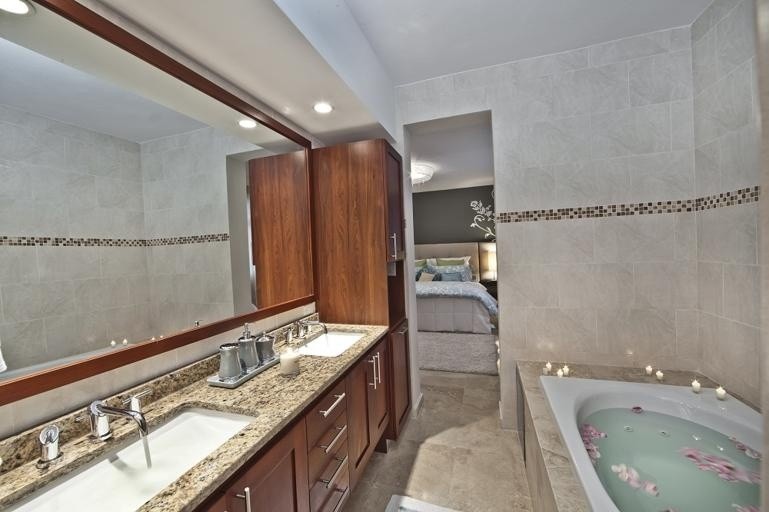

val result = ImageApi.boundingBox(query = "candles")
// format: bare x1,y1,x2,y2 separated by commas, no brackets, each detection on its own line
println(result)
715,385,726,399
656,370,663,380
542,362,570,377
646,365,652,374
692,379,701,392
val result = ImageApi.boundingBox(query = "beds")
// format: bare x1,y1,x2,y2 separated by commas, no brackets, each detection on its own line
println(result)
414,242,498,334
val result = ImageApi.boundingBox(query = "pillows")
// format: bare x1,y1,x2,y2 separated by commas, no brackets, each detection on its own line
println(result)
415,256,472,281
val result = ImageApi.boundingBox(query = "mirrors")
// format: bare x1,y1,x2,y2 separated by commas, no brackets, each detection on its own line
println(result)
0,0,319,404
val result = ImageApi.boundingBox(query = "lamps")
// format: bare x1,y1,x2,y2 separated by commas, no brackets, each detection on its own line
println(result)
479,243,497,281
412,163,436,184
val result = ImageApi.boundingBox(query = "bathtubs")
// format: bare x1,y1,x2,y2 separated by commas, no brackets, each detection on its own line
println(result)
539,375,768,511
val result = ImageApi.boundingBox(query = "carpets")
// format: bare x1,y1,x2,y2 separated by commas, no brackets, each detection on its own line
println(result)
417,323,498,376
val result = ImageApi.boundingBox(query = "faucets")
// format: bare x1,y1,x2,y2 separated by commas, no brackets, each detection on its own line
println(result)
293,319,328,340
84,398,148,444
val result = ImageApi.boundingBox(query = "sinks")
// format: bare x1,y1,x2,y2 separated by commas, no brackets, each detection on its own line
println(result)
282,326,369,358
0,400,262,512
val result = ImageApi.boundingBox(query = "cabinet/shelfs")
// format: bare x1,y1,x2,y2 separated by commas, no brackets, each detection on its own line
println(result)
140,327,392,512
248,149,314,311
311,138,413,440
